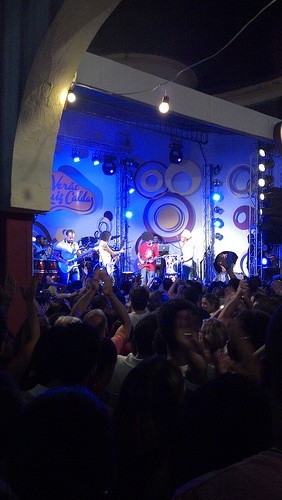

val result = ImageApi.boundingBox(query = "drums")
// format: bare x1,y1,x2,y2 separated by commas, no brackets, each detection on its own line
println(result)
46,259,58,286
33,258,48,286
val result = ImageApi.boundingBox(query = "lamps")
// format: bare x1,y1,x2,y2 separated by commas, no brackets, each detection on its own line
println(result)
212,163,225,241
169,135,184,165
71,145,117,175
257,142,276,268
123,158,136,218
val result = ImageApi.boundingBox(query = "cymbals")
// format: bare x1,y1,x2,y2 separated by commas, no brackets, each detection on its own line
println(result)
110,235,121,241
81,236,99,248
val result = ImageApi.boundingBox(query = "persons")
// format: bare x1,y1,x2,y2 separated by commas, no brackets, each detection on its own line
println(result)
0,255,282,500
178,230,194,282
53,229,82,285
153,234,160,244
137,232,160,287
98,231,125,286
32,235,48,259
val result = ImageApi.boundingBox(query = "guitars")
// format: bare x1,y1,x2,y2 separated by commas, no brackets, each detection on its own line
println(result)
105,247,125,276
57,245,102,273
136,255,169,270
